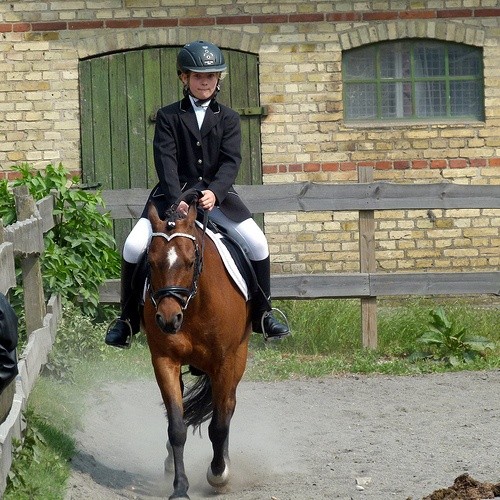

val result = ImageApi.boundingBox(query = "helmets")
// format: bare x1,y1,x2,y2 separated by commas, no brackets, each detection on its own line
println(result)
176,40,227,74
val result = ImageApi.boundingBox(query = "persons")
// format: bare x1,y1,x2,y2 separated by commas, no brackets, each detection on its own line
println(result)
106,41,287,349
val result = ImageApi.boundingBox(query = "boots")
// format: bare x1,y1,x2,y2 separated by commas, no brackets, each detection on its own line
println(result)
247,254,289,336
104,252,147,345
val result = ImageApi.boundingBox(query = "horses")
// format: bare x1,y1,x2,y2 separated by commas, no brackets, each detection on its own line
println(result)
142,190,251,500
